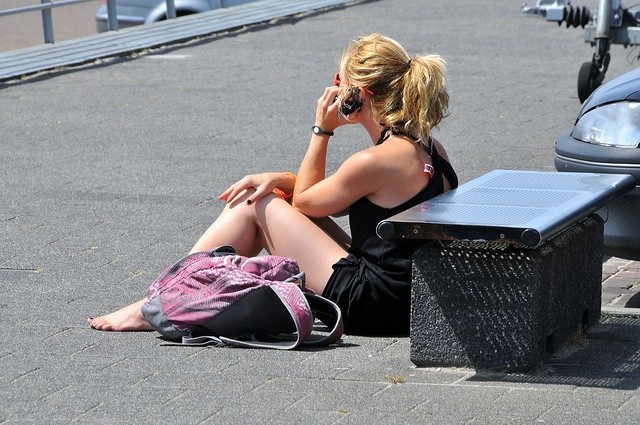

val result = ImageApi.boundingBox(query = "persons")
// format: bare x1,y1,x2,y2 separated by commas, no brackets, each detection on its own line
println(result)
86,32,459,337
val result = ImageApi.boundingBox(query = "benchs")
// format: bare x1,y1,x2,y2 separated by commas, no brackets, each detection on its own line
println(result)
374,169,635,373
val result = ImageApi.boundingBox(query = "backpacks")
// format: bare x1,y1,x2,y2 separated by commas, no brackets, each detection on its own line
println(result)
141,243,344,349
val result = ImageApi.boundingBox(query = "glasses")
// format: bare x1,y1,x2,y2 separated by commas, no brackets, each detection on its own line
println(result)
334,72,374,94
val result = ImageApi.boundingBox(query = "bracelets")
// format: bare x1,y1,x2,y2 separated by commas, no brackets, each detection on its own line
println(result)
274,172,297,197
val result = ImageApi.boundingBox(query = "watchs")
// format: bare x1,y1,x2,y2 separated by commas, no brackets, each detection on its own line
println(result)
312,126,334,136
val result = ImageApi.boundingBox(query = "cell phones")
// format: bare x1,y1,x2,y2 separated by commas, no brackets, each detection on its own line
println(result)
333,86,364,116
